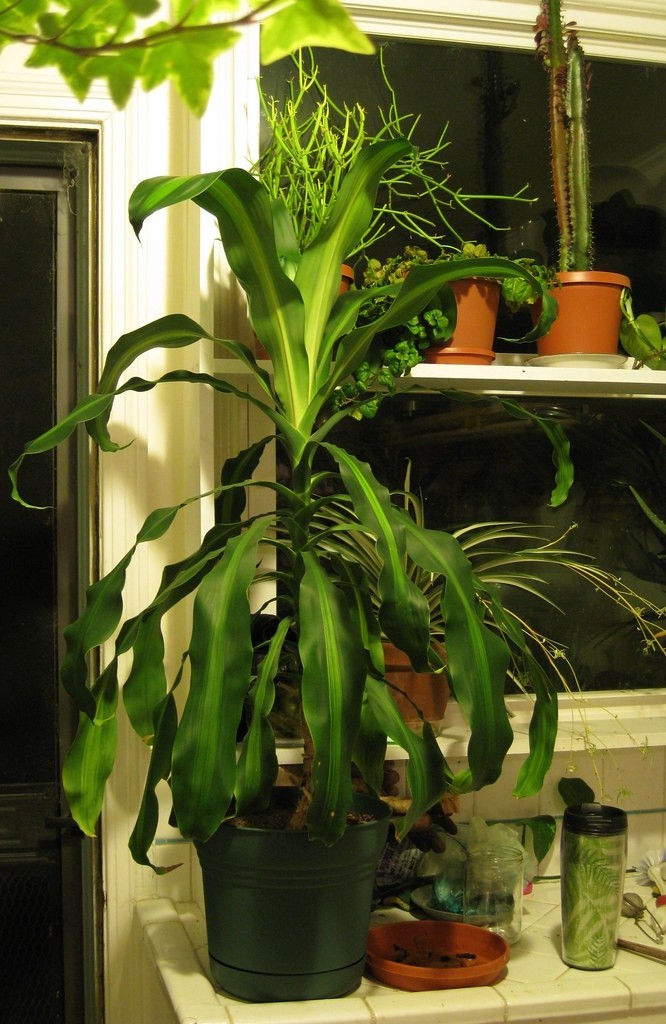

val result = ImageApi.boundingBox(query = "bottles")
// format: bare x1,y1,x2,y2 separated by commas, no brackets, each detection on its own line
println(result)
560,800,629,971
461,844,525,946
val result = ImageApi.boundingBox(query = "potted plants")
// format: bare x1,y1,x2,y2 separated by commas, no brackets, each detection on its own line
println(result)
220,457,665,741
245,42,541,419
524,0,633,369
6,134,579,1004
